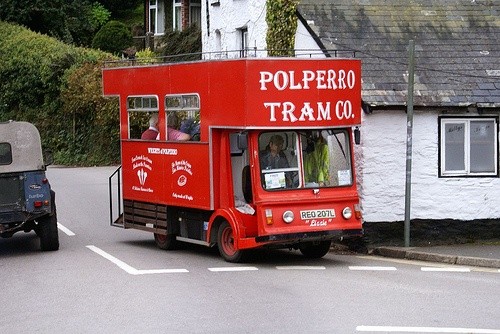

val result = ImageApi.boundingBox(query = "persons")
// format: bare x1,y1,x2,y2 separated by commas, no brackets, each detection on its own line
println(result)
259,135,298,190
141,113,200,142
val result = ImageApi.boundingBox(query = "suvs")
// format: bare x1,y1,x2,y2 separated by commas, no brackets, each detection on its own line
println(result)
0,121,59,251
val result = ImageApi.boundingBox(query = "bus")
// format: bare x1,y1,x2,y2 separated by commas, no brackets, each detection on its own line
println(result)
101,49,363,262
